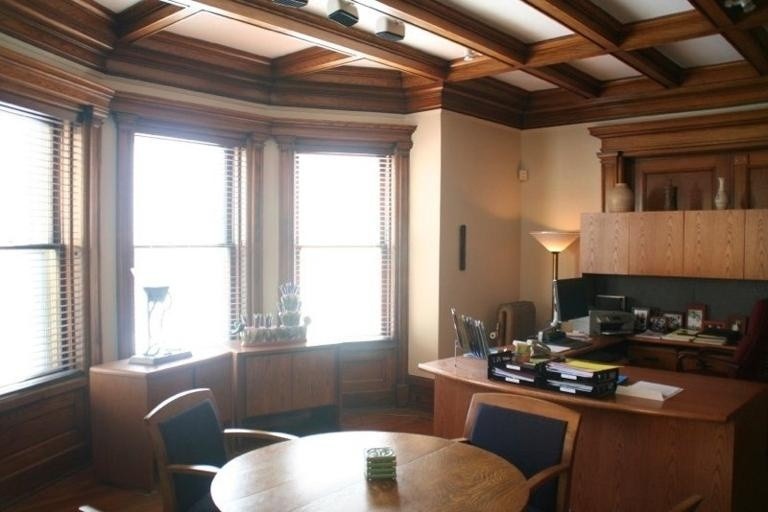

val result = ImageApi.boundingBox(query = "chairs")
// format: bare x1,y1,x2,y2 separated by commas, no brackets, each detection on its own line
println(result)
673,298,768,382
449,390,583,509
142,387,301,512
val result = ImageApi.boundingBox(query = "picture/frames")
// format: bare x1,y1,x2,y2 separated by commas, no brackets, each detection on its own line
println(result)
632,302,748,333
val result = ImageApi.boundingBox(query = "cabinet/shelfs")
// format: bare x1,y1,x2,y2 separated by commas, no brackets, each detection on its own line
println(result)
578,211,683,278
229,342,337,427
684,208,767,281
86,347,233,493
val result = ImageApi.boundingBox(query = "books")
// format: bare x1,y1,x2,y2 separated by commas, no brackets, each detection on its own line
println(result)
452,308,490,360
639,321,729,347
493,356,684,402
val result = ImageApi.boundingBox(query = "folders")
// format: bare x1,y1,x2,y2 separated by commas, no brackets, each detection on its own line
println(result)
492,367,535,383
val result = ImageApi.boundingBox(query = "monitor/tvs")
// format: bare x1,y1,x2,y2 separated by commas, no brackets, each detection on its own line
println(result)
537,276,590,345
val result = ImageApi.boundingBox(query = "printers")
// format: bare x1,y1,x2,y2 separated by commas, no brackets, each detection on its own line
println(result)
590,310,636,338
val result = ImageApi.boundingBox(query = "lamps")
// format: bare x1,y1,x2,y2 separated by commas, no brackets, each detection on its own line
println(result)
526,228,579,325
127,268,193,364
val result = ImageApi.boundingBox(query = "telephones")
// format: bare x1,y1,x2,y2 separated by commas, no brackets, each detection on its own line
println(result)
527,339,551,357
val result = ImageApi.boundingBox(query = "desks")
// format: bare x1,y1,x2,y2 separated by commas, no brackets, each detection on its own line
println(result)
208,430,533,511
416,318,768,511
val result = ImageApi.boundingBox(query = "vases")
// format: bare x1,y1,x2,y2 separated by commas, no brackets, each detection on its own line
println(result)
607,181,635,214
713,176,729,210
